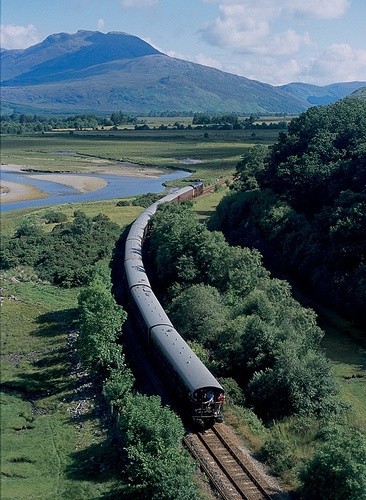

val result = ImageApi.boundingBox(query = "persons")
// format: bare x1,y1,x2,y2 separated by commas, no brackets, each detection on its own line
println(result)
218,393,225,407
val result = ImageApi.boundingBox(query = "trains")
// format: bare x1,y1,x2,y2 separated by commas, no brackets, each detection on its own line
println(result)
123,181,228,433
